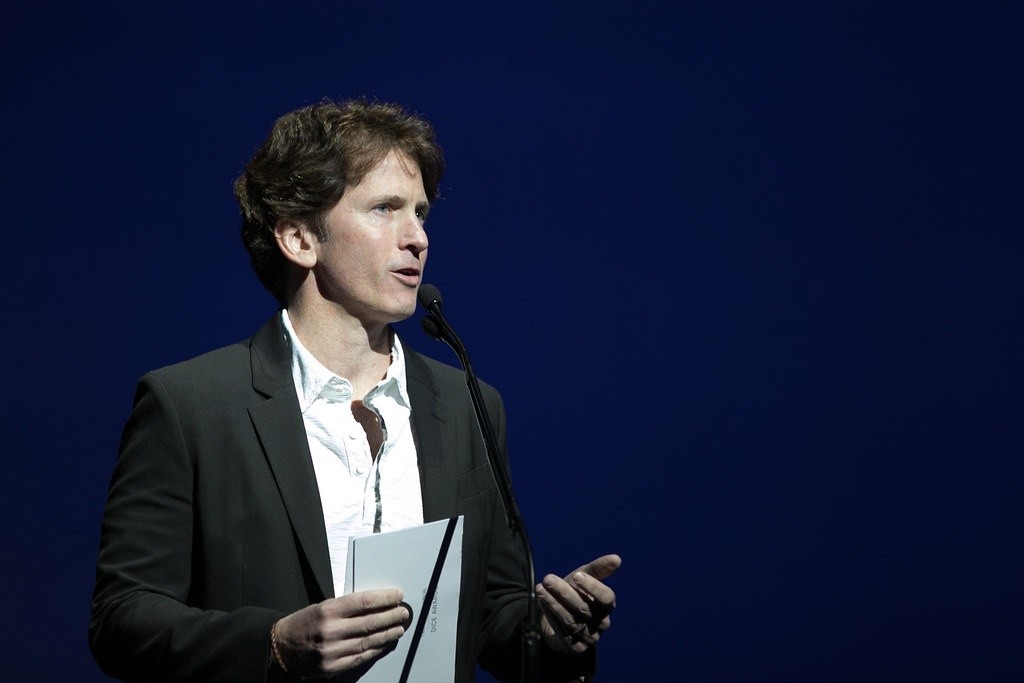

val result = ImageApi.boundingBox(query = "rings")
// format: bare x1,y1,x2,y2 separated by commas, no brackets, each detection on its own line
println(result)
575,623,587,636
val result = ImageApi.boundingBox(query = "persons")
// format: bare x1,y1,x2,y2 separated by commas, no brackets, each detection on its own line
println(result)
86,101,622,683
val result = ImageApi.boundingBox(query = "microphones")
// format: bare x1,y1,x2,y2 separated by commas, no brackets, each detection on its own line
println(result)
418,283,540,628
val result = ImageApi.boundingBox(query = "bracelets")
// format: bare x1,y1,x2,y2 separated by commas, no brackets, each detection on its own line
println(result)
271,623,287,673
